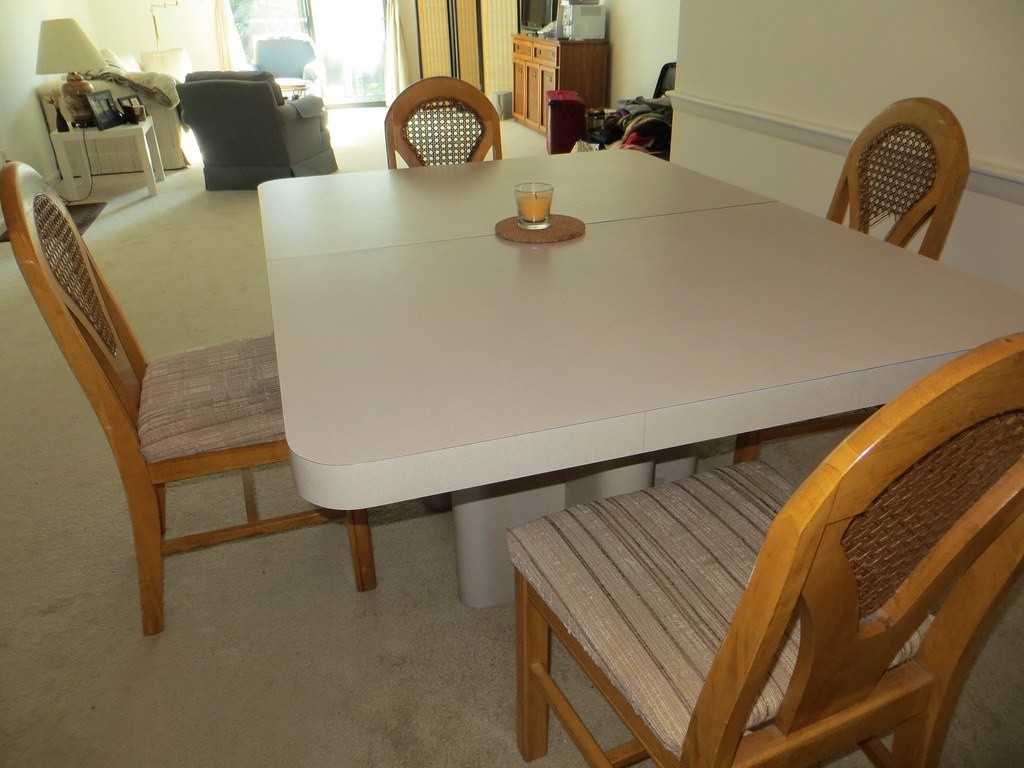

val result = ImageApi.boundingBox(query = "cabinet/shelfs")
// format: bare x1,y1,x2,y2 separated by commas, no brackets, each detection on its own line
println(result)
511,32,609,136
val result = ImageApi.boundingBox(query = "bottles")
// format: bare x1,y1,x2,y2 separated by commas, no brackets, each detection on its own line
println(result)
55,107,69,132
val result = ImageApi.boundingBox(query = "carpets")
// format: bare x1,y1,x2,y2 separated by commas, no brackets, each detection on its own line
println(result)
0,202,108,242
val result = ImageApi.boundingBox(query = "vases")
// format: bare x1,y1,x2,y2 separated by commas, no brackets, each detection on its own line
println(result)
56,108,70,132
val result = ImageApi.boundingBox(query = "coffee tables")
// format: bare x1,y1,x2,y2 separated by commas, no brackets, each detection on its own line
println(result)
274,77,308,103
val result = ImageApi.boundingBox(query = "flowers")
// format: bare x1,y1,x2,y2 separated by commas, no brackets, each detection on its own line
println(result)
44,88,61,107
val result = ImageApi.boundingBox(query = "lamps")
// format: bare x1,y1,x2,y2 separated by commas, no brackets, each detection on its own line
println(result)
36,18,108,129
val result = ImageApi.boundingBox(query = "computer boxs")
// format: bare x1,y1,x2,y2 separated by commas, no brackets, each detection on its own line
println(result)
563,5,606,40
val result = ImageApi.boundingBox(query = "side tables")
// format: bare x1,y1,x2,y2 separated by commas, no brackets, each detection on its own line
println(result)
50,115,166,198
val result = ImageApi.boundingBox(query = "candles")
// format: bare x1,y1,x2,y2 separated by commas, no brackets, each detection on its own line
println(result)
518,193,550,222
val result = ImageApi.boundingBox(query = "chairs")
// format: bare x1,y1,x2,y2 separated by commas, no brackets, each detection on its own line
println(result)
595,62,676,161
506,332,1023,768
733,96,971,463
384,76,502,169
0,162,376,637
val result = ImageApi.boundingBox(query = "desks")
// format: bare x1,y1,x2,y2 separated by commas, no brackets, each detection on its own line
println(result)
258,149,1024,606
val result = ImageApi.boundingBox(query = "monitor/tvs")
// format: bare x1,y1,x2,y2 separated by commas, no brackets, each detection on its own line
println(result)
520,0,558,37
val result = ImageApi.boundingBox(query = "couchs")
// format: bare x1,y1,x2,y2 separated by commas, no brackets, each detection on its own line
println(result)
175,71,339,191
35,47,190,176
249,34,325,113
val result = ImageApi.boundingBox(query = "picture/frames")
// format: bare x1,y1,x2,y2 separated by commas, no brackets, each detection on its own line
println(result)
87,89,123,131
117,94,149,117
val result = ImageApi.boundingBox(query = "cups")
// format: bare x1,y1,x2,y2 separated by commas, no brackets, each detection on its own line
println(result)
513,182,555,230
